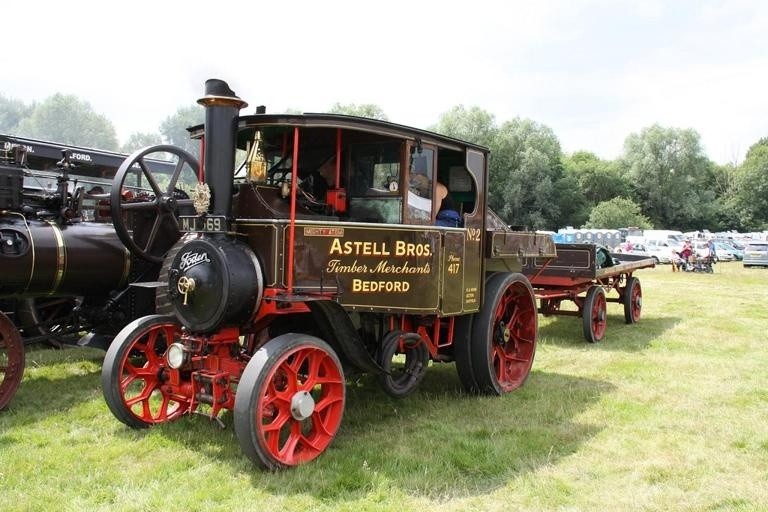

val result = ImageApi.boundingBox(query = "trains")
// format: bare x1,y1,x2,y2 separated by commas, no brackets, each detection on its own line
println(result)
99,78,557,479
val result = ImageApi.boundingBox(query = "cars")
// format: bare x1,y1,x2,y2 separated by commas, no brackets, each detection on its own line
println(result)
615,226,768,272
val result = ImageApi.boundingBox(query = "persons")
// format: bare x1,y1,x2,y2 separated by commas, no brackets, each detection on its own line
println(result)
84,186,104,195
432,182,461,227
681,240,693,272
301,138,342,187
705,239,716,274
